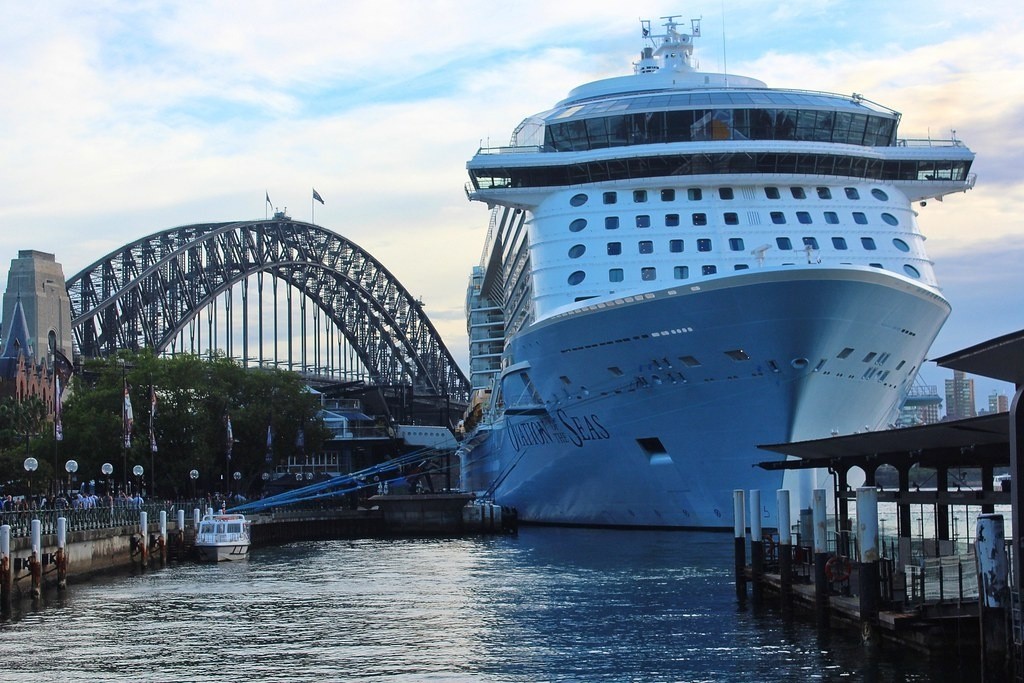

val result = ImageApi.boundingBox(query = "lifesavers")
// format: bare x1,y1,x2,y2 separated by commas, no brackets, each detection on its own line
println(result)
824,557,851,582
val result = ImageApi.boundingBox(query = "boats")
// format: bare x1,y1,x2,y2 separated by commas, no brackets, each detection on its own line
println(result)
992,475,1012,492
195,514,250,563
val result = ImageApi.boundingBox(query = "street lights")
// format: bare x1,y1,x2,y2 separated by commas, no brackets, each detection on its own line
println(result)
307,473,313,485
234,472,242,505
65,460,76,509
102,462,113,505
25,458,39,525
296,474,302,488
132,465,143,506
262,473,269,499
190,470,198,502
458,11,976,540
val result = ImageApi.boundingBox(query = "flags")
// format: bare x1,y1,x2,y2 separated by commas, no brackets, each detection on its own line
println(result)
56,375,63,442
314,191,324,204
123,387,133,447
149,386,157,452
267,194,273,210
227,417,233,458
265,426,272,461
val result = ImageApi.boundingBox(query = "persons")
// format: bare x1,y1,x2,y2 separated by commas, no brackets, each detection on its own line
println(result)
0,488,150,529
